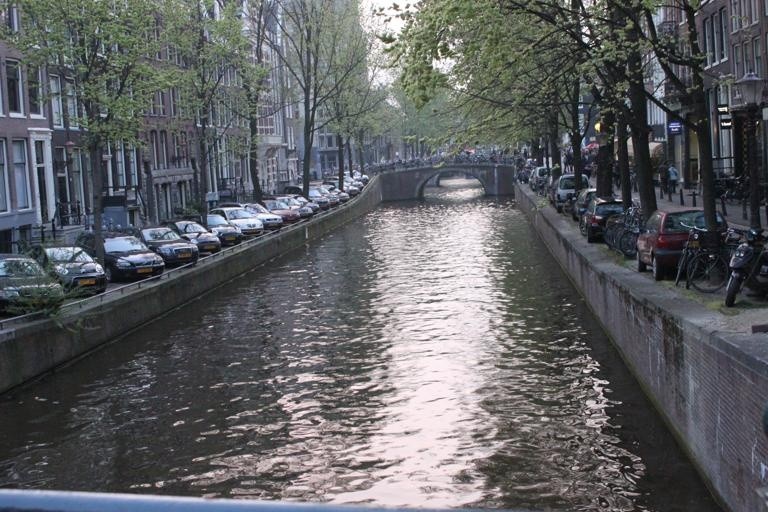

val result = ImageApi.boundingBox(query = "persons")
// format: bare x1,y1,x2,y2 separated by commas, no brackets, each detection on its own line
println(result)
658,161,669,194
563,148,638,192
669,165,678,193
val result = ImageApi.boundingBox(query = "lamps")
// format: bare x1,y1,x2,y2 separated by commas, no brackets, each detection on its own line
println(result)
55,140,74,166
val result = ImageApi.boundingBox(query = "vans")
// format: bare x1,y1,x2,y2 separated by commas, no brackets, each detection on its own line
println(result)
553,174,589,213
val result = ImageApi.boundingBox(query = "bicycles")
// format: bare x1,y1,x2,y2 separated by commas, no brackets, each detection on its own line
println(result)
604,207,639,257
715,169,767,204
674,220,747,294
562,192,578,216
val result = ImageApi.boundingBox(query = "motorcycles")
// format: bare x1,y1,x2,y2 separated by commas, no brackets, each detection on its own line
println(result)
724,227,767,306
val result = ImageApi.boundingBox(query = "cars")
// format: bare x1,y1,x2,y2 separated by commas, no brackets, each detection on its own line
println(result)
635,209,727,280
0,253,63,317
578,197,637,244
208,205,263,236
574,188,598,219
245,169,370,236
22,243,107,298
76,227,166,282
117,227,199,265
522,157,558,202
184,214,243,246
160,218,222,253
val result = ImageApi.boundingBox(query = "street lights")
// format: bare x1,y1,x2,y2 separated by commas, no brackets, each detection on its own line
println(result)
733,69,766,234
